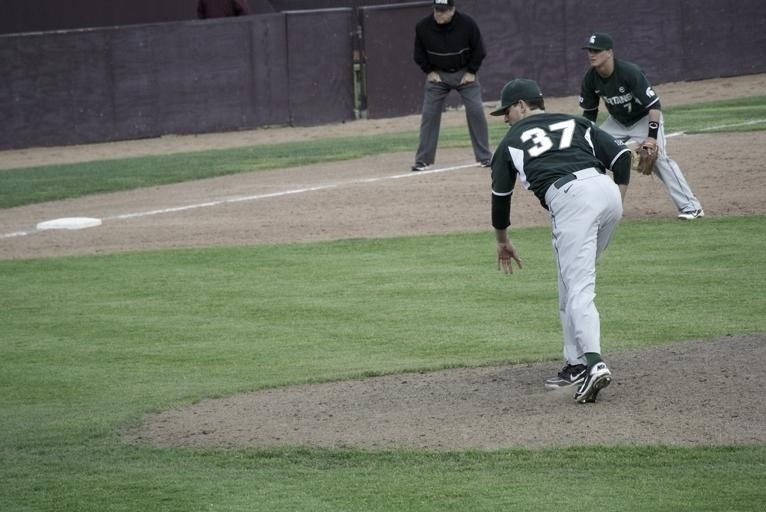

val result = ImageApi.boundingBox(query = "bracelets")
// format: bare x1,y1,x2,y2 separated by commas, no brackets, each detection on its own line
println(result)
646,119,659,139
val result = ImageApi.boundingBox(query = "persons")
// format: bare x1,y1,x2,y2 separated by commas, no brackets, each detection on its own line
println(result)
411,0,493,172
487,76,635,404
577,31,708,221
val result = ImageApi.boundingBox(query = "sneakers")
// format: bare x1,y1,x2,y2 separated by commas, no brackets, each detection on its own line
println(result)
575,360,612,404
677,208,705,221
412,161,432,170
543,362,588,392
480,161,491,167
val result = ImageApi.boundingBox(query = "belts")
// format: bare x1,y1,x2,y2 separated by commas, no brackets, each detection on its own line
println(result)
553,167,603,190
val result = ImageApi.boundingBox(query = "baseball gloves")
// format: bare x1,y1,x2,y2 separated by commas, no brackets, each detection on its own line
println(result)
635,137,658,175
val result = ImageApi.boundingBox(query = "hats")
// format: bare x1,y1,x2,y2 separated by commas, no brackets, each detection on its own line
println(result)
581,33,614,50
431,0,454,6
489,78,543,117
